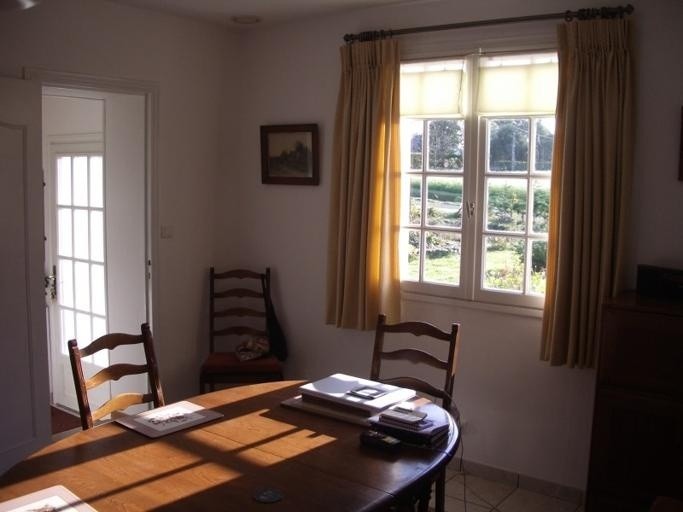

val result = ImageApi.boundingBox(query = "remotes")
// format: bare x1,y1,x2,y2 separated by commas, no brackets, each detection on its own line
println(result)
359,428,399,449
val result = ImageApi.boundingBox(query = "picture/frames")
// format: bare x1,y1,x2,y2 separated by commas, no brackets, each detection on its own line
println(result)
260,124,320,185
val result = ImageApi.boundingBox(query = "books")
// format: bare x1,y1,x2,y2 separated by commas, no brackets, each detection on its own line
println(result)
280,372,450,450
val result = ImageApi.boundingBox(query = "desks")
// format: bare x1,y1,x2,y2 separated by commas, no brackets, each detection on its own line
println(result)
1,379,458,511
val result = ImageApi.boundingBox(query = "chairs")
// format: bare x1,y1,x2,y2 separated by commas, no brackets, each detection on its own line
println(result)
67,323,165,430
370,313,460,512
198,265,283,393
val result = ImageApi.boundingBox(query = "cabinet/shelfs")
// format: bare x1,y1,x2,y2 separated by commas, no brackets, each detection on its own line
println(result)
584,290,683,512
